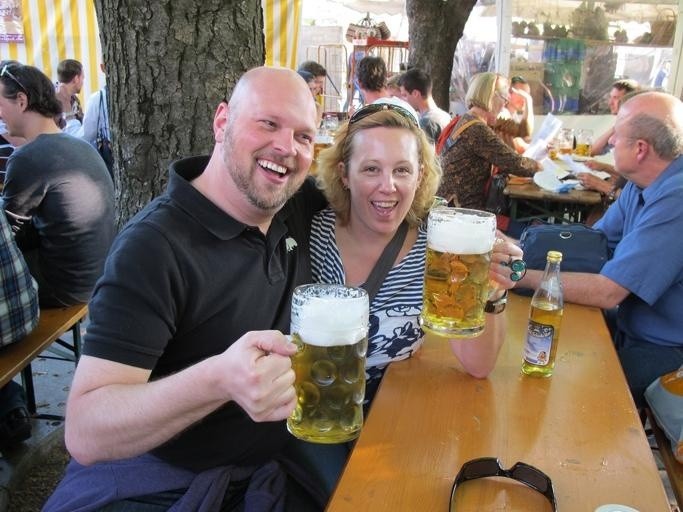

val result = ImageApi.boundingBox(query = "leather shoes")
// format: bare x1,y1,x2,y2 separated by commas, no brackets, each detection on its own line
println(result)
0,407,32,448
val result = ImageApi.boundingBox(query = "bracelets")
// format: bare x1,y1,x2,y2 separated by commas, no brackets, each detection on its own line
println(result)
608,187,618,200
484,290,509,314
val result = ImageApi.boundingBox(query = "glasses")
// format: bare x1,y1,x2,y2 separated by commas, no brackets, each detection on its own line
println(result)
1,62,29,95
500,95,509,107
340,103,419,163
512,76,523,83
449,457,557,512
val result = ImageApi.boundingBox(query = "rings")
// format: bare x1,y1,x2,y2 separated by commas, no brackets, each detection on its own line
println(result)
500,254,527,281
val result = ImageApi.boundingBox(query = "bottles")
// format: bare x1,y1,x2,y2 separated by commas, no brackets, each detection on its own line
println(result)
520,250,566,378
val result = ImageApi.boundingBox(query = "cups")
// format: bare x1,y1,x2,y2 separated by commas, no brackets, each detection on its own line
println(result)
310,135,336,176
546,139,560,161
276,281,372,446
419,205,506,339
558,127,575,155
577,127,592,157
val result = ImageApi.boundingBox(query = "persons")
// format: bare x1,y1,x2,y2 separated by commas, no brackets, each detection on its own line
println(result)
1,200,39,345
299,57,451,144
436,73,539,239
2,57,115,444
343,50,373,112
290,103,523,497
40,67,330,512
577,79,644,226
512,91,683,408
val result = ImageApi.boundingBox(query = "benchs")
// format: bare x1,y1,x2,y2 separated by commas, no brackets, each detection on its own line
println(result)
0,303,88,385
639,400,682,511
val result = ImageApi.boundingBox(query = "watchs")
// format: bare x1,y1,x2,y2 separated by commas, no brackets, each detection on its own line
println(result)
61,112,74,120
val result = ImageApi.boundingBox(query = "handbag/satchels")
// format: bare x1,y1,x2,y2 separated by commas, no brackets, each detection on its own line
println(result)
95,90,115,184
484,170,511,216
512,222,614,298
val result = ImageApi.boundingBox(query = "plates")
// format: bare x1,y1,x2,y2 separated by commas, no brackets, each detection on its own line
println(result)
594,502,640,511
531,169,565,192
